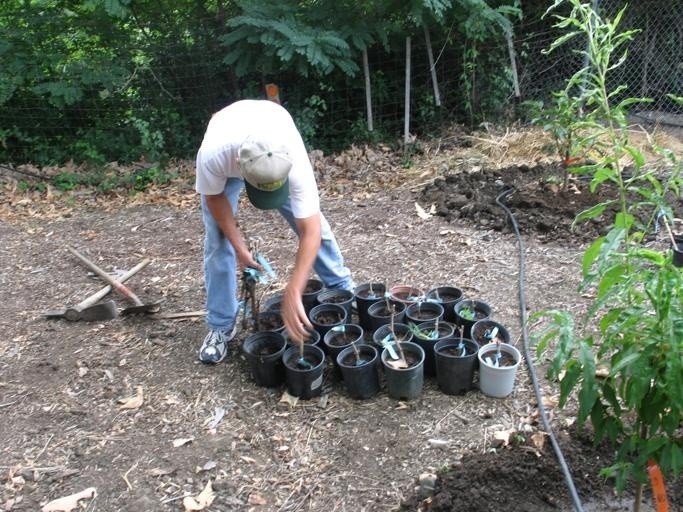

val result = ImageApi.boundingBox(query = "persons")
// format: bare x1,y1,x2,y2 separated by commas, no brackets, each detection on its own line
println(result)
193,98,360,365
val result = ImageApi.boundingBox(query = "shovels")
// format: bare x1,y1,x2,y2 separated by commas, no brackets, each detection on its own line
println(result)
67,245,165,315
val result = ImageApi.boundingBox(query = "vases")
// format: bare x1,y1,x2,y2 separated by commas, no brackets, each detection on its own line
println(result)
242,278,524,402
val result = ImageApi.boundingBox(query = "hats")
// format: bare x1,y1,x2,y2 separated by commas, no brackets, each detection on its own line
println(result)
235,133,292,211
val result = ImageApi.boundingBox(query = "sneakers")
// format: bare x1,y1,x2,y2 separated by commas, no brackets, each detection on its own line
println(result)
197,320,238,365
342,281,363,316
